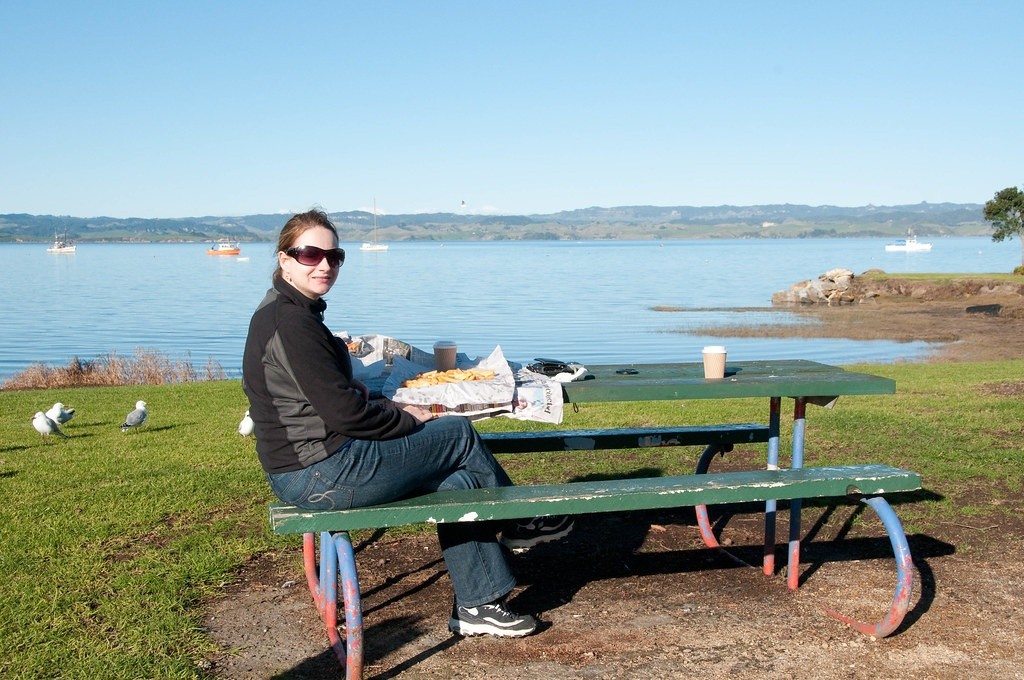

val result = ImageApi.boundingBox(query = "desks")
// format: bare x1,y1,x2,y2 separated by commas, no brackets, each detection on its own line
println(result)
345,358,896,589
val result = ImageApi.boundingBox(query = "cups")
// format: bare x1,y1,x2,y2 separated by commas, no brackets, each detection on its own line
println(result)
433,340,457,373
702,346,727,379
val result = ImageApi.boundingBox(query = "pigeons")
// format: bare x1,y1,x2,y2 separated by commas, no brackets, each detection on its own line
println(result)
119,400,148,432
45,403,75,430
238,410,256,437
32,411,67,442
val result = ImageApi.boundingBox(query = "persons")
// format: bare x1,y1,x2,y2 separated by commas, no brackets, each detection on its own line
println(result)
242,209,575,637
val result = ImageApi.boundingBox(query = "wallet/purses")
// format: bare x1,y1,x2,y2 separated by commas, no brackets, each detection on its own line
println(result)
532,358,574,377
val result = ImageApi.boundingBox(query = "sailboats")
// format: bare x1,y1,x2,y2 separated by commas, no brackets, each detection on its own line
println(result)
360,196,388,251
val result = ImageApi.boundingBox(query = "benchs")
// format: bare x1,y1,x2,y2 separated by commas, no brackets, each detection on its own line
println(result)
265,463,924,680
476,423,772,553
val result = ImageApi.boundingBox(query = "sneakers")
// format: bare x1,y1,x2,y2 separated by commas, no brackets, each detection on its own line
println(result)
448,604,537,639
500,515,575,552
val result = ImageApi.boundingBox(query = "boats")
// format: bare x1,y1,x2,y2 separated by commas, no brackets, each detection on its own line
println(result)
47,226,76,253
207,235,240,256
885,227,934,252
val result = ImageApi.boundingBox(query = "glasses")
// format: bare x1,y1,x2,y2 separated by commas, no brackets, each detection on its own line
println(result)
285,245,345,269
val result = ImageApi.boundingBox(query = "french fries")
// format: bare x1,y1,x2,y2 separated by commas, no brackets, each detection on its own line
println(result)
401,368,496,388
